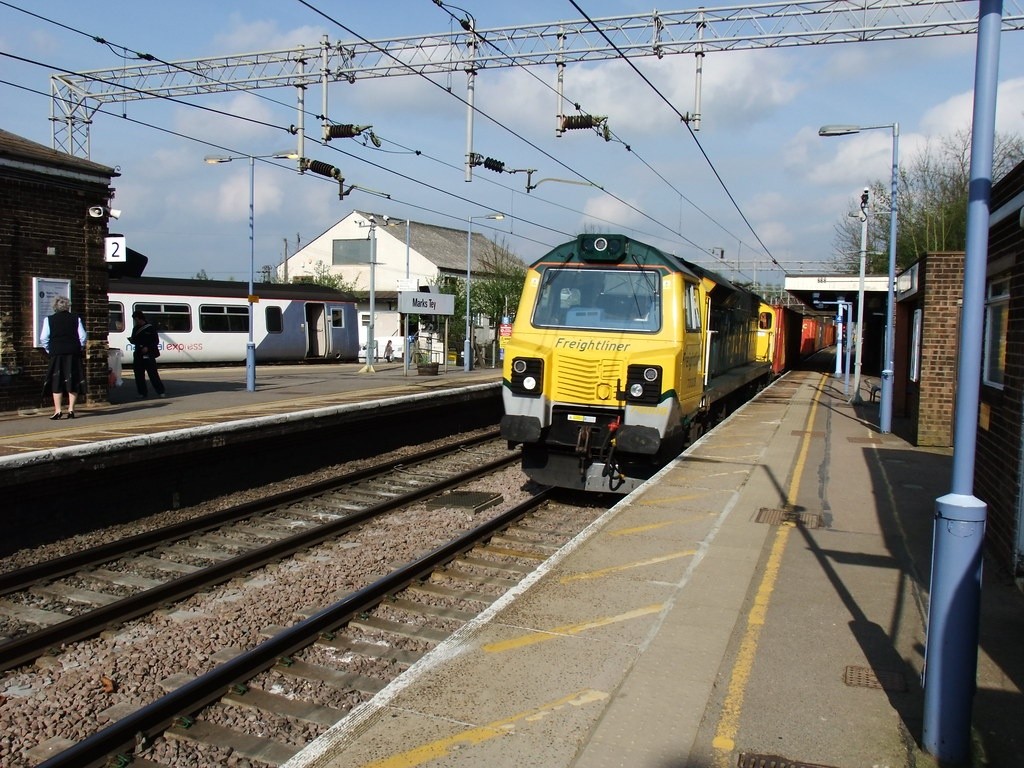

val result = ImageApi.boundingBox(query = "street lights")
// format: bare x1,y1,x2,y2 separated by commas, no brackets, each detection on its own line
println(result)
203,149,299,392
464,213,506,372
389,218,408,378
818,122,899,436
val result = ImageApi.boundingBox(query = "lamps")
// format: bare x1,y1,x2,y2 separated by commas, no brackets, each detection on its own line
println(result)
89,204,122,221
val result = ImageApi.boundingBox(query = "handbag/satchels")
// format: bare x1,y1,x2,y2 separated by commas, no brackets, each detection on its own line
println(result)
147,344,160,360
389,351,394,361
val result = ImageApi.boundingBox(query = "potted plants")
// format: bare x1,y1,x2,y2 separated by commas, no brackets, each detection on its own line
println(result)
417,355,439,376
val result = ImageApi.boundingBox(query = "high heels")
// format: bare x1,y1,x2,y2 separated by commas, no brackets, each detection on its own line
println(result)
67,411,74,419
49,411,63,420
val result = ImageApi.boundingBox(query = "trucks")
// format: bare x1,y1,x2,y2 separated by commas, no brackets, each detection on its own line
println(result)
358,335,404,364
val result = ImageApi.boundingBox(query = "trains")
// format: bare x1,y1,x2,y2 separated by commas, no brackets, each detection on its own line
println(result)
105,277,360,368
501,231,837,495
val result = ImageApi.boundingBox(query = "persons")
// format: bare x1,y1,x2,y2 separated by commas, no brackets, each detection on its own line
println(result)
383,340,395,363
128,310,166,399
40,296,87,420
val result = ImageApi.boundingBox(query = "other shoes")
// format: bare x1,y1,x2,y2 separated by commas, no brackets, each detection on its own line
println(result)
136,395,147,399
160,393,165,398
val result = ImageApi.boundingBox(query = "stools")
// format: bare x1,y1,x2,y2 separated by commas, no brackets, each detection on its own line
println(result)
870,384,880,403
